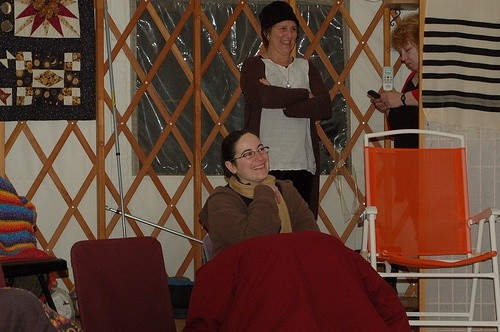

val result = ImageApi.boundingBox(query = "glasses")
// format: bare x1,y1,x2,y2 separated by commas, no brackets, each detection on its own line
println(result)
231,146,269,161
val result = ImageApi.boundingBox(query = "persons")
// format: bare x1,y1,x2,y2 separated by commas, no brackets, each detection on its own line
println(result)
240,0,333,222
368,15,419,149
197,129,321,257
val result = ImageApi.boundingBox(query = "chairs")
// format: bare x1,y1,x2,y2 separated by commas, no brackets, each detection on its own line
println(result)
71,237,176,332
357,129,500,332
0,175,67,314
267,170,319,218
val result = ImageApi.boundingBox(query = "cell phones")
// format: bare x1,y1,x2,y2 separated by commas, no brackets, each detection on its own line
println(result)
368,90,381,98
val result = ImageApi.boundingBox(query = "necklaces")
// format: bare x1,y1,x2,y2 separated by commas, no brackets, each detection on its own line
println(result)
267,54,291,88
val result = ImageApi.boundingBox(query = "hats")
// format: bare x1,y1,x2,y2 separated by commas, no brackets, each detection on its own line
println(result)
259,1,300,32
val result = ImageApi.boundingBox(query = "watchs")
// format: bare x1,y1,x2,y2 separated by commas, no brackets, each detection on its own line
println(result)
401,93,407,105
